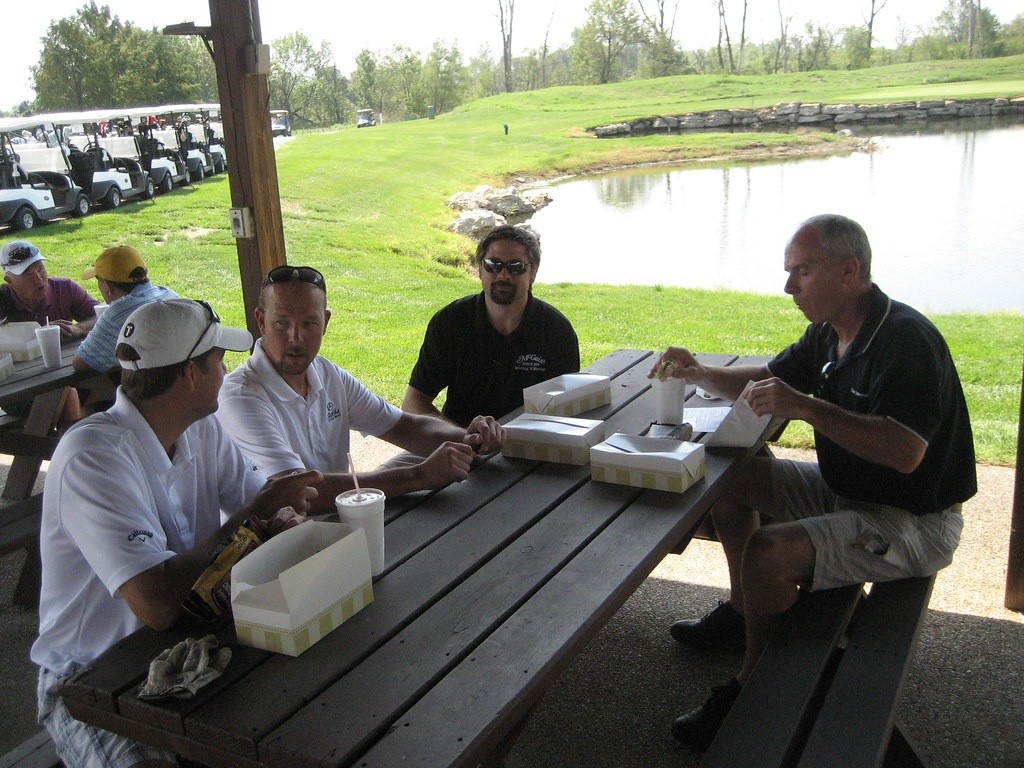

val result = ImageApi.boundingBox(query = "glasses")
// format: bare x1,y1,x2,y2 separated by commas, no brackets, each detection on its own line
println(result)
1,246,39,266
182,299,221,377
260,266,327,295
480,257,531,276
815,361,837,400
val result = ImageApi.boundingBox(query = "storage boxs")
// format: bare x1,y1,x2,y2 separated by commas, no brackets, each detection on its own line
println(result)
523,375,611,418
0,321,44,362
591,429,706,493
229,520,375,656
502,413,604,467
0,351,15,383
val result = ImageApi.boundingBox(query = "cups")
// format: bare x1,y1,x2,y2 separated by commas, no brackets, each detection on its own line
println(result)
35,325,62,367
652,378,686,425
94,305,108,319
334,488,386,578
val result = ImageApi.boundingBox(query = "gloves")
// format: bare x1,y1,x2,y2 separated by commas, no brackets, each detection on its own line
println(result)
137,634,233,711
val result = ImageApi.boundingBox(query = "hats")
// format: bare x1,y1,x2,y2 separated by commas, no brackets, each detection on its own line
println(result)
115,297,254,371
81,245,147,282
0,240,48,276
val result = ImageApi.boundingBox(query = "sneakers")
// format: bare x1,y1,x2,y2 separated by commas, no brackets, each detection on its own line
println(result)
671,676,742,748
670,599,746,648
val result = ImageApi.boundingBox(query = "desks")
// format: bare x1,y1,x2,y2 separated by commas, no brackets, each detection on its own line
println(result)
0,334,123,500
58,347,795,768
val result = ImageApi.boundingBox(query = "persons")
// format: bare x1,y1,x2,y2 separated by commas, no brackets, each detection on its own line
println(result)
217,264,506,515
39,297,324,768
0,240,100,419
72,246,183,372
401,224,581,460
648,213,980,741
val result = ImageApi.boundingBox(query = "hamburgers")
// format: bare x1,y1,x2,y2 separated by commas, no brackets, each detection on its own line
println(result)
653,360,680,382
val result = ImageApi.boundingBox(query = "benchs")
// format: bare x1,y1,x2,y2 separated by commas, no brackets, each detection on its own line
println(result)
0,122,224,189
0,493,42,608
703,565,939,768
